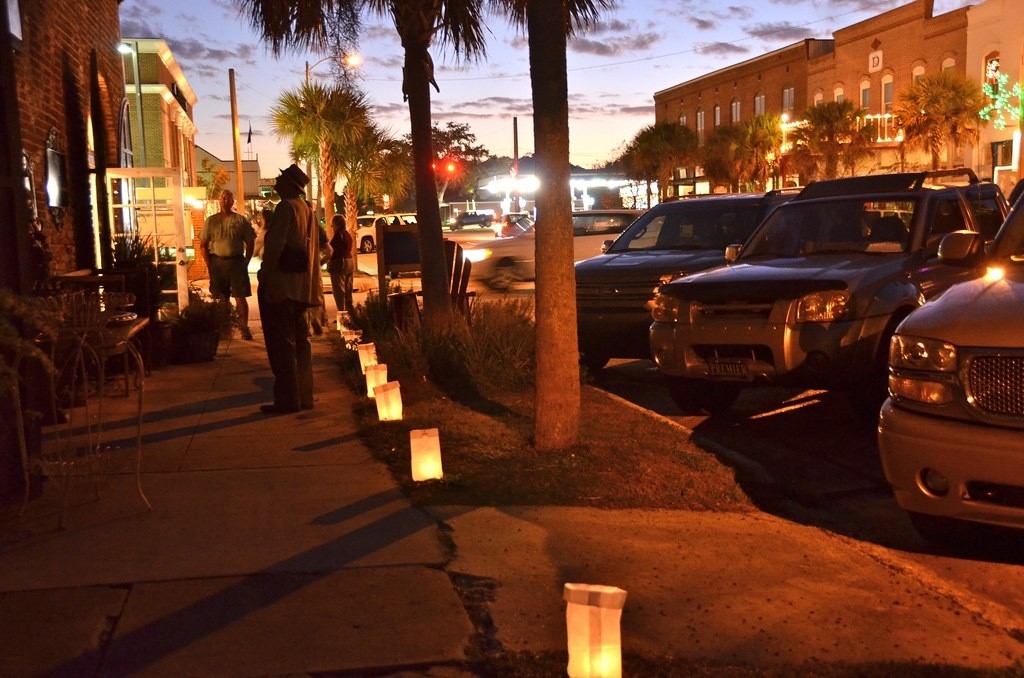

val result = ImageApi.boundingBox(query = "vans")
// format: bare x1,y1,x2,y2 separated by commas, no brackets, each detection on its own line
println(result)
357,213,417,255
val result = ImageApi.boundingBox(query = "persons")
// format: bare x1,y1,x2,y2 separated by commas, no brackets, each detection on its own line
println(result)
197,189,256,340
252,201,353,339
256,164,320,413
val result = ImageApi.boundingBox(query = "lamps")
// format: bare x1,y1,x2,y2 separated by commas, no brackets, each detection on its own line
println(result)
365,364,388,398
336,311,348,331
410,428,443,482
357,342,378,375
372,380,403,421
562,582,628,678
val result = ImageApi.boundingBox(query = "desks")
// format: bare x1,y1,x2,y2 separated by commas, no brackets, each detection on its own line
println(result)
57,318,151,399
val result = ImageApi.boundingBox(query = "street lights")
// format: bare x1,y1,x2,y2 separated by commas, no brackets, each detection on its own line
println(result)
117,42,148,188
781,114,788,150
305,53,364,203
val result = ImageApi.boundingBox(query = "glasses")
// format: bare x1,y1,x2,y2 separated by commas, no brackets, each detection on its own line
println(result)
217,195,231,200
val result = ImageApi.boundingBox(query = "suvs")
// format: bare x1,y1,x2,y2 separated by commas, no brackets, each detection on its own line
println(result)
575,189,803,372
648,168,1015,438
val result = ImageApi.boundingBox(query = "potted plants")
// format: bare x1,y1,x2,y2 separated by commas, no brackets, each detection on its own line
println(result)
179,301,243,363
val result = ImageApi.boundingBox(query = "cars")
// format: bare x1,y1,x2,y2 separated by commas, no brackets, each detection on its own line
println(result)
446,208,668,292
877,189,1024,543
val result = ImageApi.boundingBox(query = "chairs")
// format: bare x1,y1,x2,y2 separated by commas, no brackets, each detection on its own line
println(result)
871,216,910,243
828,222,865,243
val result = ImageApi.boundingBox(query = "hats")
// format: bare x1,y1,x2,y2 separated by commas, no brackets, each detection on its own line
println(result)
280,162,310,196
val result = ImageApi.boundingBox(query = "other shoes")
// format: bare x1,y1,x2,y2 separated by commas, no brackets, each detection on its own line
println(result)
240,326,253,341
311,318,323,336
258,401,300,414
